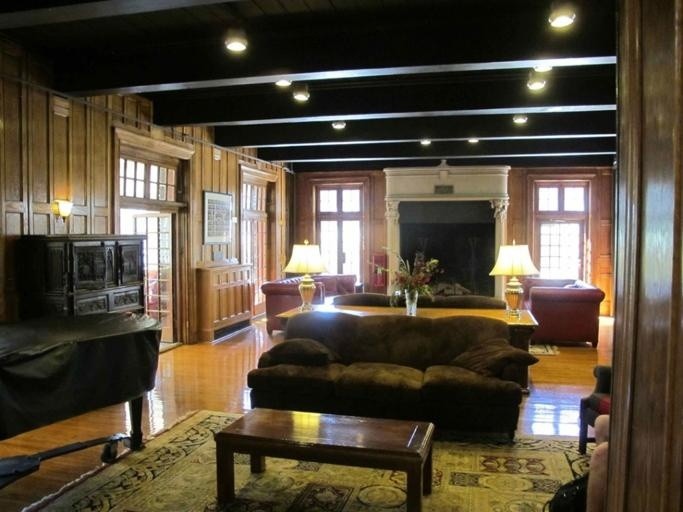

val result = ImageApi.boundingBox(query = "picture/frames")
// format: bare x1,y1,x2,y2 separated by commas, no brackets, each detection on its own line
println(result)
203,191,234,245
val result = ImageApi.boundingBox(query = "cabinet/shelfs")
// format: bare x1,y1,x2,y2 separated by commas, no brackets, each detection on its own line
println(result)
196,262,254,341
6,232,148,316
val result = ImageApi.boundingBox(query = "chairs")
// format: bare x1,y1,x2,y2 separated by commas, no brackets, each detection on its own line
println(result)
579,366,612,455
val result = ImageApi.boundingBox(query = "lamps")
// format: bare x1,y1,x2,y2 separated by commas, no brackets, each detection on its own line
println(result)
489,245,541,322
283,238,330,313
331,121,346,130
512,114,528,124
224,29,249,52
548,7,576,28
55,200,74,220
293,84,310,101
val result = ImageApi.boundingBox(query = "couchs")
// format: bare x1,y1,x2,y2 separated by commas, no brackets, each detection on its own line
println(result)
261,274,357,336
332,293,507,310
247,311,539,440
520,278,606,348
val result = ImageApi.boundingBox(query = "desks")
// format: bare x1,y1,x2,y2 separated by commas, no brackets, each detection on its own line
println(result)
0,316,163,493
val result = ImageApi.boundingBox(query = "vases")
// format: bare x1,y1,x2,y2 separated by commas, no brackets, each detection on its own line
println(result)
405,289,418,317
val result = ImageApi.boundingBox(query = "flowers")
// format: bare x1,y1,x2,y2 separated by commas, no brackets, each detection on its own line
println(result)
366,247,440,315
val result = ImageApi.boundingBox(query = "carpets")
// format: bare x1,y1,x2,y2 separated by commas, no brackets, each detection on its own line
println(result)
18,411,613,511
